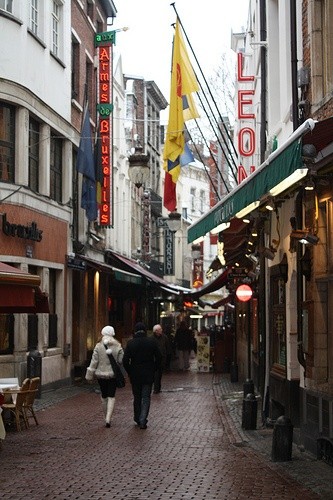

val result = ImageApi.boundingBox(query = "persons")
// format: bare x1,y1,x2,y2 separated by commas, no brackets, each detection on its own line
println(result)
85,325,123,428
123,323,163,430
149,320,198,394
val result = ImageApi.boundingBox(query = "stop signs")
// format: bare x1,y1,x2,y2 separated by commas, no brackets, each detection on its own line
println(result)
236,284,252,302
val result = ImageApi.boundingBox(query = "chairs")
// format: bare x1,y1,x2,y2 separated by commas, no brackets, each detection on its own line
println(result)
0,377,40,433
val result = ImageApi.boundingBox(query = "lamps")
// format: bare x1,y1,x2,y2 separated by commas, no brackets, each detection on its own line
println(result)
235,231,258,267
166,208,182,233
242,215,250,224
191,244,201,260
128,139,151,190
304,183,314,190
265,201,275,211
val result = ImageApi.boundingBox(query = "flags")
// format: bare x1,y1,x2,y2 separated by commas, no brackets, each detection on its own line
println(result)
163,18,202,214
76,100,104,221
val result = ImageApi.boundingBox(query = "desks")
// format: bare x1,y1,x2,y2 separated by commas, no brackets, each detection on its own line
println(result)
0,378,26,430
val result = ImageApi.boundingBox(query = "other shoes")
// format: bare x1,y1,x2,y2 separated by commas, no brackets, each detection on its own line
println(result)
106,421,111,428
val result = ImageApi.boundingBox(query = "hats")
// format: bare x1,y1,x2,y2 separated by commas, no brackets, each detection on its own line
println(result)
134,322,147,334
101,325,115,336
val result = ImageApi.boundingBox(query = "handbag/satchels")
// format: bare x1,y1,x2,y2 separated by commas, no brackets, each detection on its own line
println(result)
104,345,126,388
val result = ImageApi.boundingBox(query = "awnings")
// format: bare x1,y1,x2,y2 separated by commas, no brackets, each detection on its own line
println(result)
0,262,51,314
79,254,142,286
186,118,316,245
112,253,192,296
188,266,236,309
